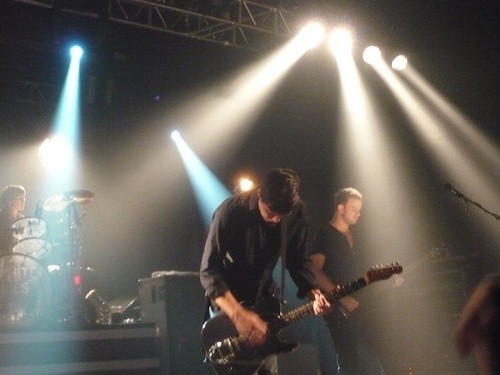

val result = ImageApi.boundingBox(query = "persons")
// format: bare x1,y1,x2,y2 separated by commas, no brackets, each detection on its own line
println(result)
200,168,331,375
309,187,404,375
0,185,29,258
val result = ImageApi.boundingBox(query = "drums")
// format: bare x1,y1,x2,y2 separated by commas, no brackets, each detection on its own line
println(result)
8,215,53,260
0,252,56,332
50,262,97,325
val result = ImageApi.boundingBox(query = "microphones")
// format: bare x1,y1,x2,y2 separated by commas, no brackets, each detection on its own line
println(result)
443,183,464,199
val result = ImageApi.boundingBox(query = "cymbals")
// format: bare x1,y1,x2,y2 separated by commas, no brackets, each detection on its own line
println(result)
66,189,94,199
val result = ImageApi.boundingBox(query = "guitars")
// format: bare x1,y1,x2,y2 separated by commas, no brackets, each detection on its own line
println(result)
199,260,404,375
323,242,452,327
